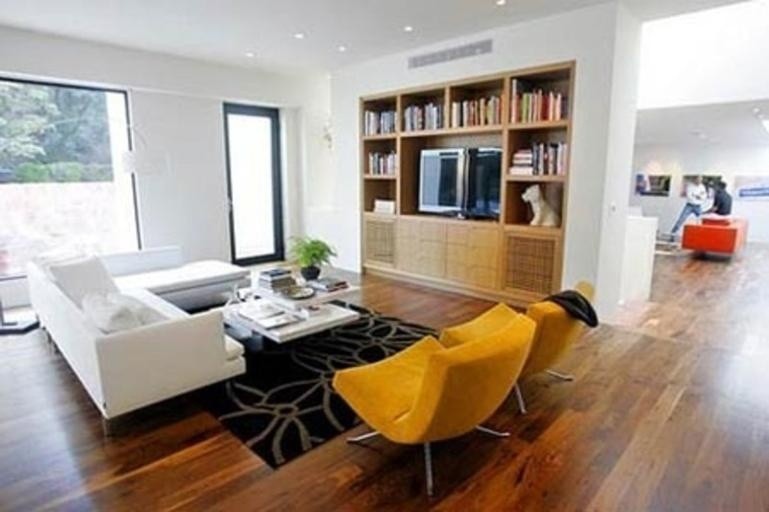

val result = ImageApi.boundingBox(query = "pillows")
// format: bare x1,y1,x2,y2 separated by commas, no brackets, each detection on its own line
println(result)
39,252,141,334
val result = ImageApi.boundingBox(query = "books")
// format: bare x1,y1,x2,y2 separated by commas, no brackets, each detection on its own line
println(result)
237,265,352,332
361,78,570,217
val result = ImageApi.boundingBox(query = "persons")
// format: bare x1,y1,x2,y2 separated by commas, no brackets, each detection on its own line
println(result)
668,172,710,243
696,181,733,227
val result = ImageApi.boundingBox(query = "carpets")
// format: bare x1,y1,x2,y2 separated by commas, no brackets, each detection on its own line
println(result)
189,297,441,471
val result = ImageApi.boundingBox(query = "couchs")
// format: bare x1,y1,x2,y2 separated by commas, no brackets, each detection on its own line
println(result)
27,243,258,437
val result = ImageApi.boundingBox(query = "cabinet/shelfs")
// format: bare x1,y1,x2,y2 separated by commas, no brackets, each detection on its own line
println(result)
358,60,578,309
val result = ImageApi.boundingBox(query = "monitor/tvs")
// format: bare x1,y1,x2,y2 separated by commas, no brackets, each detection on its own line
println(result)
418,147,503,220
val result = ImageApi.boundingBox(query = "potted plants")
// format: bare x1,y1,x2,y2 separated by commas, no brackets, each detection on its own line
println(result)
288,234,334,282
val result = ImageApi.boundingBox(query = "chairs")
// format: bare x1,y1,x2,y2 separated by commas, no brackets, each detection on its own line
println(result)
435,281,595,418
681,216,747,260
330,312,539,502
